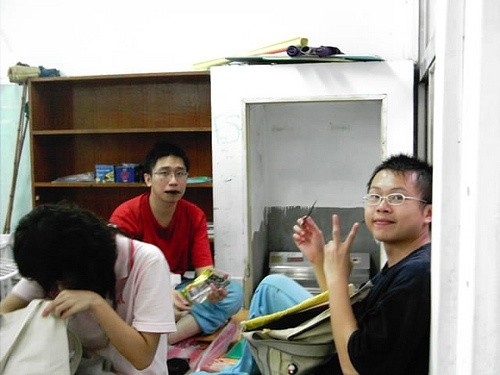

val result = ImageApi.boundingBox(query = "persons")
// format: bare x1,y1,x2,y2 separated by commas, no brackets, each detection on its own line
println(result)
190,155,432,375
109,142,243,346
0,203,176,375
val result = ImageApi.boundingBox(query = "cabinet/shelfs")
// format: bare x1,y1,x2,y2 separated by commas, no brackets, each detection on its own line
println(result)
28,70,213,261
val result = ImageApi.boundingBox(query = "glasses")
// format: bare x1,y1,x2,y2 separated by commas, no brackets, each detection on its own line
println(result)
150,170,191,181
363,193,429,206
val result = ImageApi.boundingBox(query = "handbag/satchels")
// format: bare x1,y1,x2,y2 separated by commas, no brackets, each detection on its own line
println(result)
0,299,83,375
242,281,374,375
